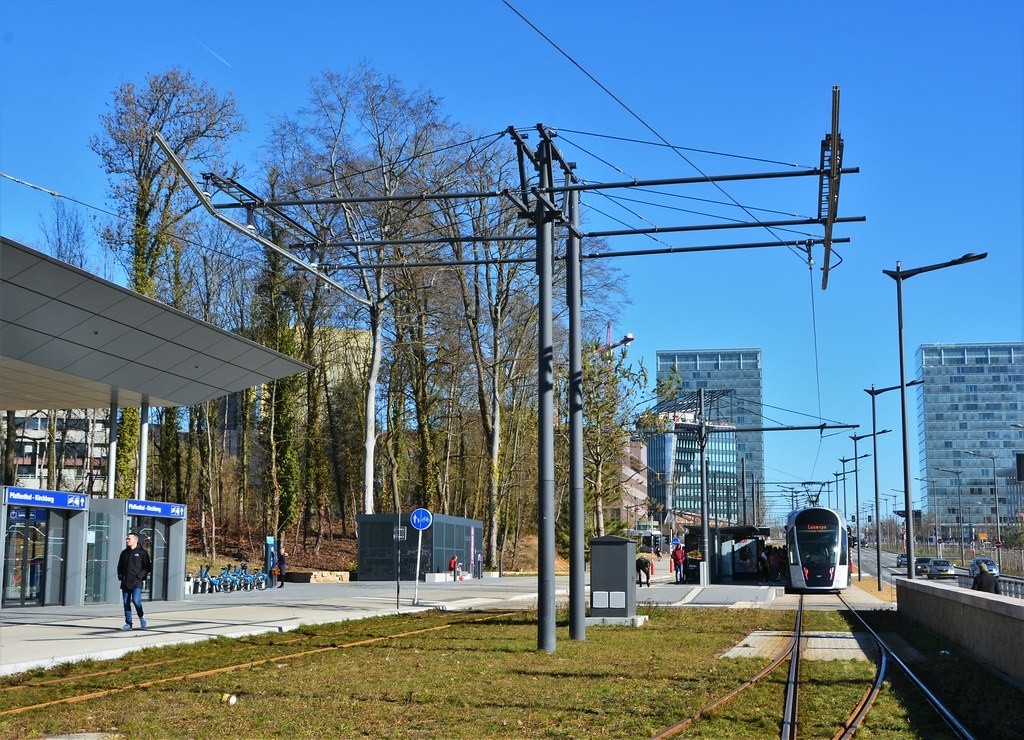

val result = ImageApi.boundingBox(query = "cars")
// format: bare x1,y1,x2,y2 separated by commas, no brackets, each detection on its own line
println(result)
915,557,932,576
969,557,999,578
896,553,916,567
926,558,957,579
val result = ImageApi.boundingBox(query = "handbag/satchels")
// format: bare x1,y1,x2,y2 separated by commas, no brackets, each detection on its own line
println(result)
272,568,280,575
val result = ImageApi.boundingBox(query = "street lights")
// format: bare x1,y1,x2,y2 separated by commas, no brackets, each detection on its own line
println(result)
934,467,965,567
849,493,897,553
839,454,872,519
977,502,987,549
964,451,1002,574
658,507,678,553
826,477,849,509
832,469,860,509
914,478,939,558
777,485,794,511
882,252,990,579
863,380,926,591
848,428,893,582
623,503,646,538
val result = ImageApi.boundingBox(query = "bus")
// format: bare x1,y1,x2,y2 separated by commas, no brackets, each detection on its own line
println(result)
783,481,848,594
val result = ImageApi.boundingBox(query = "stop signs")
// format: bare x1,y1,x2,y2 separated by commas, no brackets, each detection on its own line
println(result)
994,541,1003,548
937,539,943,544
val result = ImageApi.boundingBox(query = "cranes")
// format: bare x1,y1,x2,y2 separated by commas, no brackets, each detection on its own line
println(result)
562,323,635,431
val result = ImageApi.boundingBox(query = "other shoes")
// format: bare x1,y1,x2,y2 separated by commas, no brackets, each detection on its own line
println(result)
120,623,133,631
140,615,147,629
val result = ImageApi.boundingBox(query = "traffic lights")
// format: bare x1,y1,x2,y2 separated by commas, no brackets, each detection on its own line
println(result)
665,535,670,545
654,535,659,548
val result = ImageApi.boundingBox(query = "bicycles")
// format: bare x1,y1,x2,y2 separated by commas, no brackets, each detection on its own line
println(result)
195,563,270,594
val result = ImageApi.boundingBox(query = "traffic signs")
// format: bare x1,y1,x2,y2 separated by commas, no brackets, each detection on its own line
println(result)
127,499,185,519
7,487,87,511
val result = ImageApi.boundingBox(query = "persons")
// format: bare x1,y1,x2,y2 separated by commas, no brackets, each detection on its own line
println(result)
670,543,688,583
117,533,153,630
275,547,286,588
654,546,663,558
449,555,465,581
970,564,1003,596
761,545,788,581
